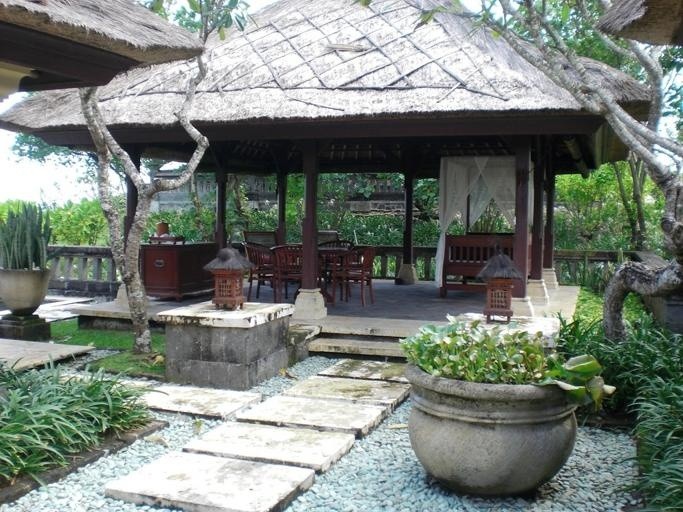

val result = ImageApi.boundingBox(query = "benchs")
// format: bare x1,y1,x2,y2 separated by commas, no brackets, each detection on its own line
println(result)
438,231,515,299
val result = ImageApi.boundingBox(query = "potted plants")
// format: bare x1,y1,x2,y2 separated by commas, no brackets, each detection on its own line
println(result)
397,312,618,499
0,203,57,317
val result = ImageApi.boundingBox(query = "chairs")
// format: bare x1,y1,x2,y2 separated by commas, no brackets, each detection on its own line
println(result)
242,231,375,308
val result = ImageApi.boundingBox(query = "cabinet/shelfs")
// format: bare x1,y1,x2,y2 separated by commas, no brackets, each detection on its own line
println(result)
137,243,218,302
